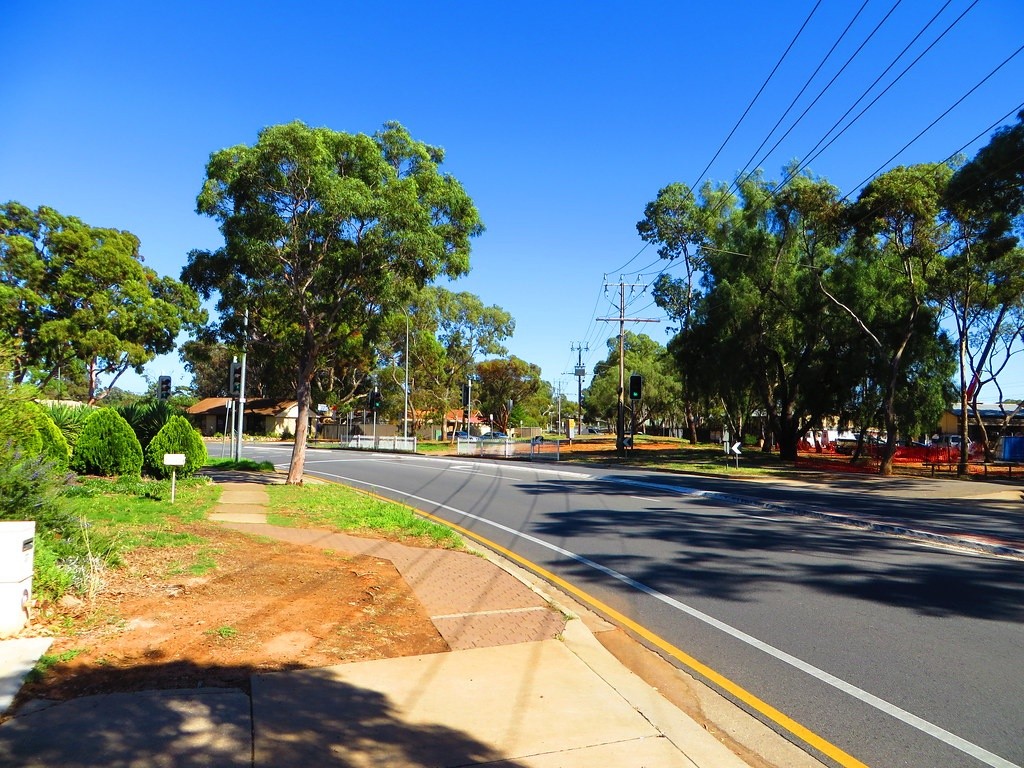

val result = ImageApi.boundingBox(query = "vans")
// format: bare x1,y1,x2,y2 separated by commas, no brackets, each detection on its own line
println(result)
932,434,973,448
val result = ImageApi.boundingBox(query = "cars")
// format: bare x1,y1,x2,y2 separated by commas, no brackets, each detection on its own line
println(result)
479,432,508,440
893,440,927,449
437,430,480,441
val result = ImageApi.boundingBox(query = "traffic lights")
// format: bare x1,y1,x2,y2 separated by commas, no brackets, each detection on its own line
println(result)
158,375,171,403
370,392,380,410
459,384,469,407
629,375,641,399
229,363,242,396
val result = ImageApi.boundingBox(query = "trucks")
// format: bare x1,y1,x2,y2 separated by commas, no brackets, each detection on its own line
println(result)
799,429,861,450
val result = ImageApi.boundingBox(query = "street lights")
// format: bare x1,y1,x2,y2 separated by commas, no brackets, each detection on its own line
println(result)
379,298,409,443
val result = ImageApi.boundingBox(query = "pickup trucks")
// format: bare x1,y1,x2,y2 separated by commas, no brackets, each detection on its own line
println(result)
834,436,887,455
